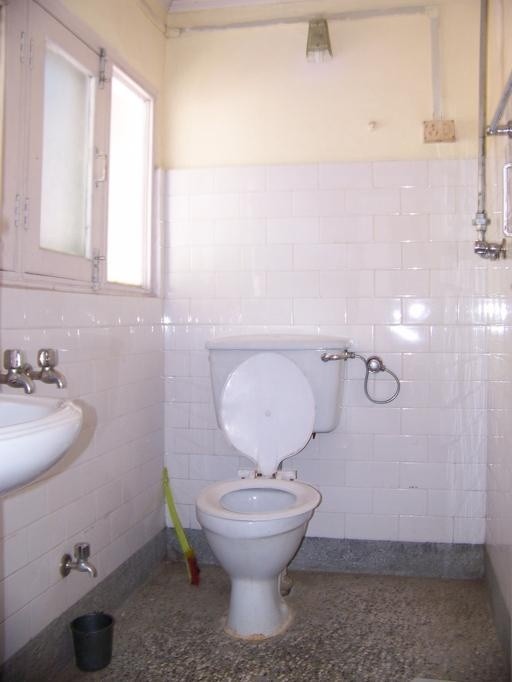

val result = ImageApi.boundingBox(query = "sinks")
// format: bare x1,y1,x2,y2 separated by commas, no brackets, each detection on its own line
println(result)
0,396,83,496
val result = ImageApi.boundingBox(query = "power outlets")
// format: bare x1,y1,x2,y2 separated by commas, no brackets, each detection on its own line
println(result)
423,120,454,142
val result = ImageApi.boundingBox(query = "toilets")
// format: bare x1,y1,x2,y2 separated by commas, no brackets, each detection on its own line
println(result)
196,335,348,637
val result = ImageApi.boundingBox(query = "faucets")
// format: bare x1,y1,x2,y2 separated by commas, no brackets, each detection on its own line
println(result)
26,349,67,390
0,347,37,395
62,545,99,580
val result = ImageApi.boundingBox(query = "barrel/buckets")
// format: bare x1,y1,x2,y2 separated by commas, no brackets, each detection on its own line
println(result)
70,612,114,672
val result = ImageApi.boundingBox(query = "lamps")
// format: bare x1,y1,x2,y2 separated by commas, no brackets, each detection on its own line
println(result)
306,19,331,61
